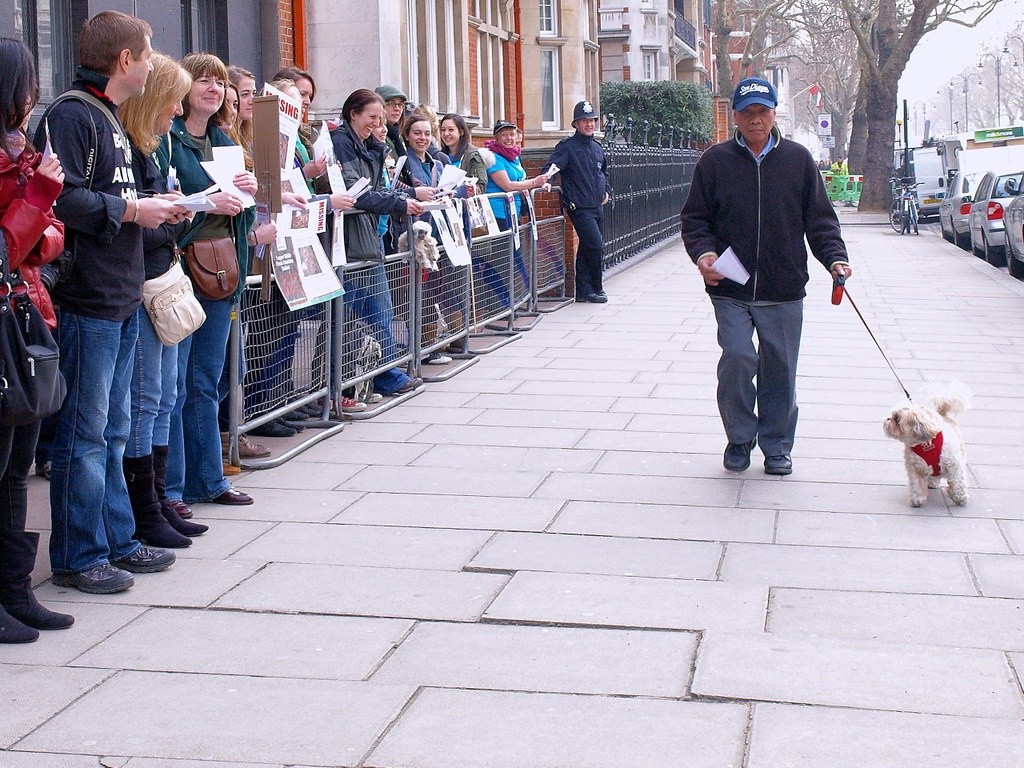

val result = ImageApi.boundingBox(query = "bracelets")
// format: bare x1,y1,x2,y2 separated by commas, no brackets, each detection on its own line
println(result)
133,200,140,223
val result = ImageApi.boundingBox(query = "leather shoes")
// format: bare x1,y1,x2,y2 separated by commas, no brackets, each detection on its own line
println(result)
722,438,756,472
763,453,792,474
576,289,607,303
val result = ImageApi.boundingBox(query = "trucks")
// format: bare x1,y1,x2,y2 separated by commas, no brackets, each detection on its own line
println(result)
936,125,1024,191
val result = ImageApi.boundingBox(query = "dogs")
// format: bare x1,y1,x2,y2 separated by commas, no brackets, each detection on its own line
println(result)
883,401,969,507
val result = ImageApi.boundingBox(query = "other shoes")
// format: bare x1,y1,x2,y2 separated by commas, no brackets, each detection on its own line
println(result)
219,328,468,460
212,488,254,506
171,500,194,519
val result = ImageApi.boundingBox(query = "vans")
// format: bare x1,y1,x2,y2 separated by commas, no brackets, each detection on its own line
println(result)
906,148,946,222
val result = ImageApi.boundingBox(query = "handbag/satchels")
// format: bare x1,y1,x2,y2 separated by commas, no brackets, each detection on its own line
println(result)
141,248,206,349
183,235,244,301
0,230,67,427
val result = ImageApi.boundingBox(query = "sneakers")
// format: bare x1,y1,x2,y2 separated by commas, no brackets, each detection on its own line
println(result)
50,545,175,594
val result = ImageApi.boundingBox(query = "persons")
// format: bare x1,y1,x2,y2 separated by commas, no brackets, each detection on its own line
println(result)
31,12,178,596
510,126,524,153
117,53,209,549
433,112,485,350
480,119,553,319
214,64,452,475
0,35,75,644
156,54,254,518
539,101,614,303
680,77,853,474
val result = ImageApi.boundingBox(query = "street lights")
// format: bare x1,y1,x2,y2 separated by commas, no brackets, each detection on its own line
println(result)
896,36,1023,147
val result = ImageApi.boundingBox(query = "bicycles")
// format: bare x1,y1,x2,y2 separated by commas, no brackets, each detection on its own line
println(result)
888,177,925,235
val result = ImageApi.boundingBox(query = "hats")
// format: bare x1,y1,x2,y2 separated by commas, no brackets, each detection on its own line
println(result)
731,77,778,112
373,85,407,101
491,120,515,135
570,99,598,129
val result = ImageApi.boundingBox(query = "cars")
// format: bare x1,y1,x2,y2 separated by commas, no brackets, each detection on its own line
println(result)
936,169,988,248
960,169,1024,268
1003,172,1024,282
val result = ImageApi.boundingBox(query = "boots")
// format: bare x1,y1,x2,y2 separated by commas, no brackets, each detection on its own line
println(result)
123,445,208,548
0,530,74,643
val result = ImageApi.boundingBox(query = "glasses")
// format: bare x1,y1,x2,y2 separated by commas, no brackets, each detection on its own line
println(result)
195,78,228,88
